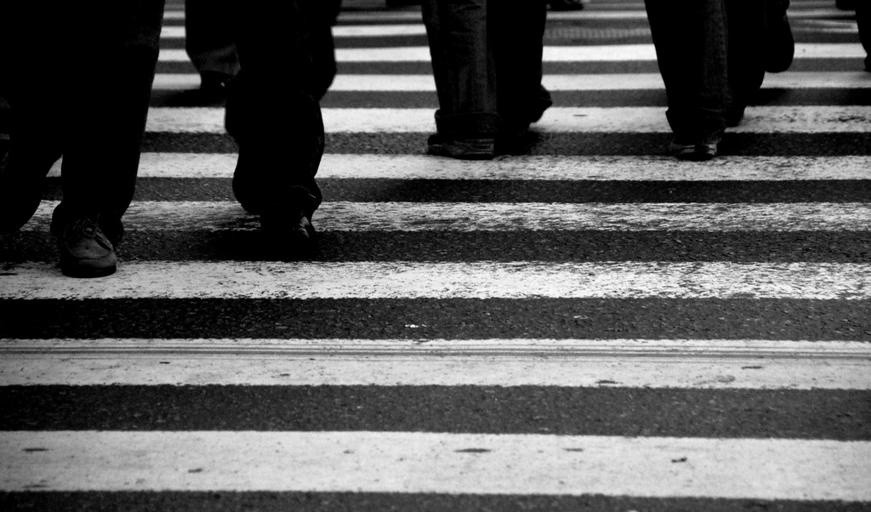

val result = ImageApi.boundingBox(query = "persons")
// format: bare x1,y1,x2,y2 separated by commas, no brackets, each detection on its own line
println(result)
0,1,166,279
852,0,871,72
181,1,344,241
420,0,554,161
639,2,798,159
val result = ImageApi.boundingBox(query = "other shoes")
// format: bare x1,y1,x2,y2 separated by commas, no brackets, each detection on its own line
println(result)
427,132,496,160
671,135,719,161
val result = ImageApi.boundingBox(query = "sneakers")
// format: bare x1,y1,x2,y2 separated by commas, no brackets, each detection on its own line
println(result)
261,208,321,251
54,221,123,277
